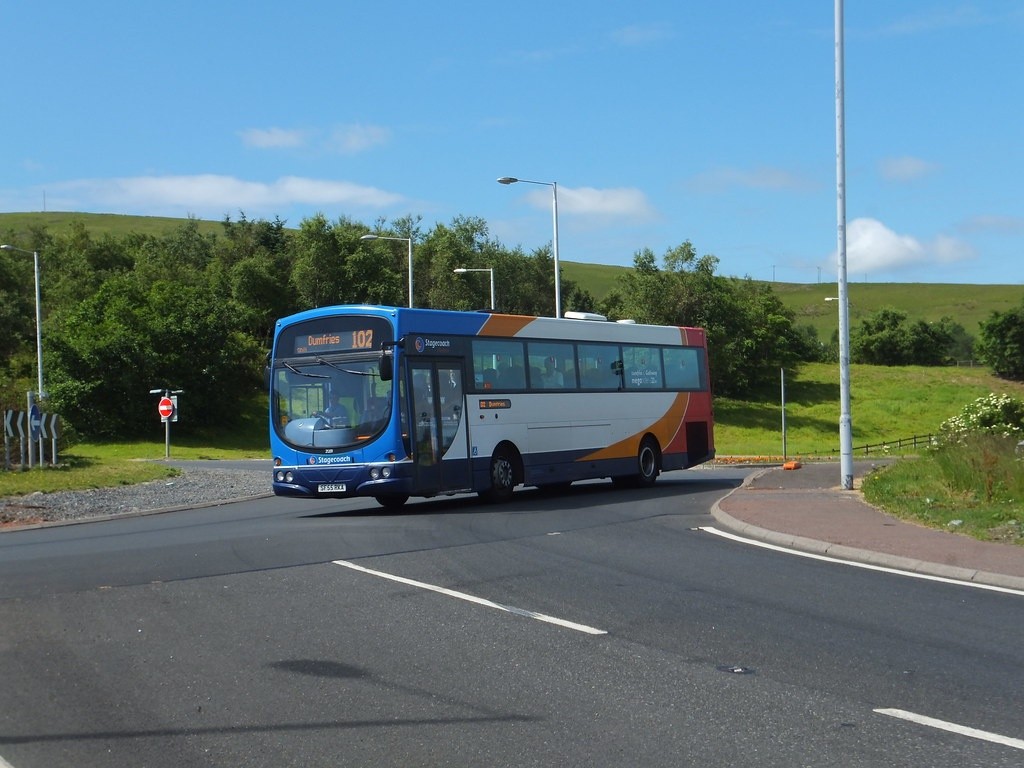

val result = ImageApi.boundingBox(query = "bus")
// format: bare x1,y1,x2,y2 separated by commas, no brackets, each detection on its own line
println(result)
263,304,716,509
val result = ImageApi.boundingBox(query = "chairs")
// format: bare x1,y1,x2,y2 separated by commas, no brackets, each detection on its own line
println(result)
411,363,684,427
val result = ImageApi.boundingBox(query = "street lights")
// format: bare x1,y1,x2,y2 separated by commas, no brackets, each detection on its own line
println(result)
360,234,413,309
0,243,44,469
453,268,496,310
497,177,561,318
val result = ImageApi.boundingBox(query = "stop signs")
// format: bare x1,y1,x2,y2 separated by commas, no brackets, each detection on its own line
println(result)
158,398,174,417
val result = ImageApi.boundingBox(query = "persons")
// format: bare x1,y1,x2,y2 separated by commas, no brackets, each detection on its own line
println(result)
384,370,462,451
481,357,659,387
312,391,348,428
